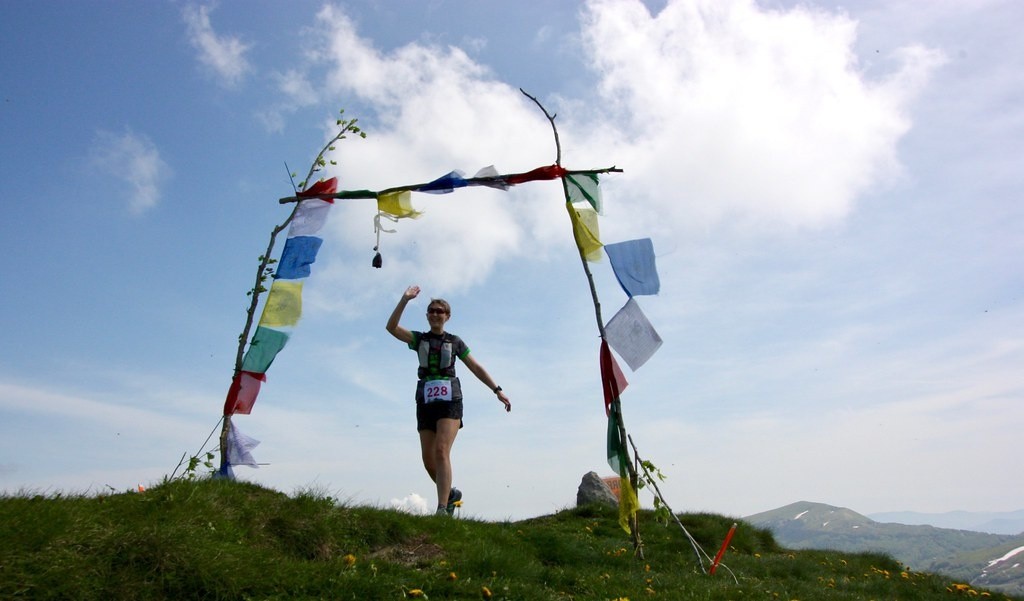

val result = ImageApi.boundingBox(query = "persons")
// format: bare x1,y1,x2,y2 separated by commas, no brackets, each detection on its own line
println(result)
385,285,510,517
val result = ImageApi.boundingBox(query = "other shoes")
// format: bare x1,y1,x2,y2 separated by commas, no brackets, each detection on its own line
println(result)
447,489,462,517
436,504,448,516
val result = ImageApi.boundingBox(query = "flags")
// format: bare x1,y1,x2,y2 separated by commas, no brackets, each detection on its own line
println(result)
605,402,629,479
300,164,565,220
563,173,604,216
604,238,660,298
600,340,629,416
618,474,640,536
567,202,603,264
602,297,663,372
220,199,331,482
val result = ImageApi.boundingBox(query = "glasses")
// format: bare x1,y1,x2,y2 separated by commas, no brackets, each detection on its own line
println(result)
427,308,444,314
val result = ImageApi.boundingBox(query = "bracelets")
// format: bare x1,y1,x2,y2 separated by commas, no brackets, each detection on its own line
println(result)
493,386,502,393
